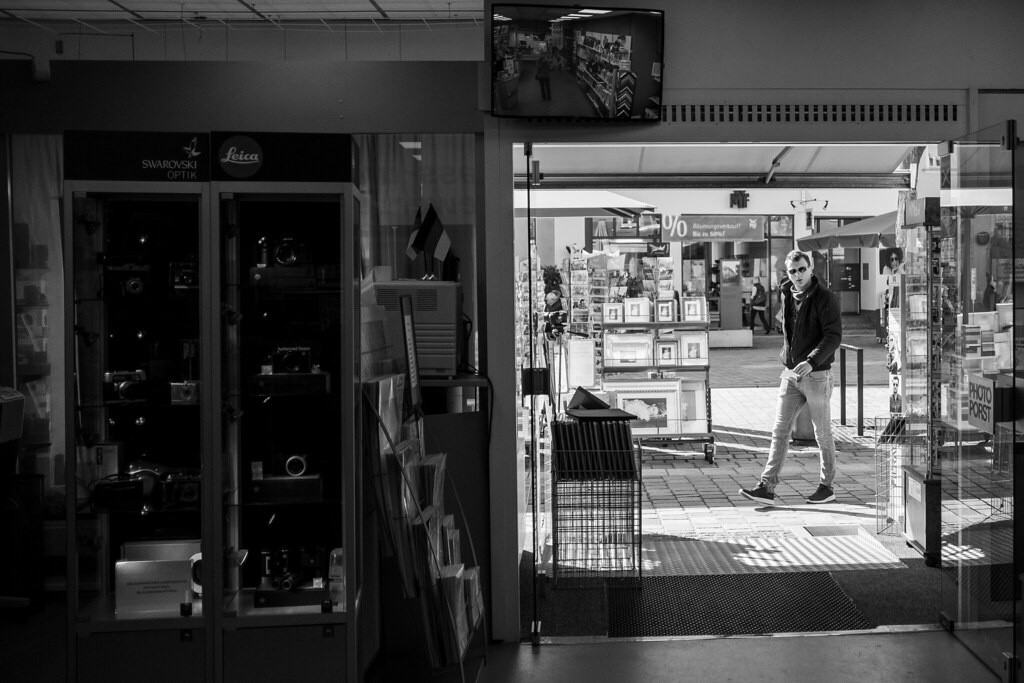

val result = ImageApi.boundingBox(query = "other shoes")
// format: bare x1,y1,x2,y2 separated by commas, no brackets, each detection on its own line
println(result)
765,330,770,334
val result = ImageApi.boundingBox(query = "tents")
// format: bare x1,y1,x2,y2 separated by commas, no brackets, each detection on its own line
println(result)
797,210,896,292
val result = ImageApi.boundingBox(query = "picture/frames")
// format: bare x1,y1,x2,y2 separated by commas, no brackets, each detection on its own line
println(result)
602,296,709,438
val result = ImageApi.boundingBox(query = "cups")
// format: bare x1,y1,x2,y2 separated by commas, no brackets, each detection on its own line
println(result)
328,548,344,580
329,581,345,603
260,365,272,375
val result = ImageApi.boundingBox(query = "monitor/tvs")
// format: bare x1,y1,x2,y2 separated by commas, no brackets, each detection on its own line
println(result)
484,0,664,123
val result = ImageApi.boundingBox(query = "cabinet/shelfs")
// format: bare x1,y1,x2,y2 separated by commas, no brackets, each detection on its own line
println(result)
886,275,962,522
63,130,379,683
600,303,715,463
361,372,487,683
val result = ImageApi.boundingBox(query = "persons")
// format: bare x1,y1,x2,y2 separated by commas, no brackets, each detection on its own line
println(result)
981,270,1013,315
623,399,667,422
737,250,843,507
889,376,902,413
877,280,890,344
492,32,630,102
749,275,771,336
882,247,907,275
544,290,569,313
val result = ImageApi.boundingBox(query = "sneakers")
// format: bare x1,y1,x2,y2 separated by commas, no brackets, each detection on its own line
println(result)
739,482,775,505
806,484,836,503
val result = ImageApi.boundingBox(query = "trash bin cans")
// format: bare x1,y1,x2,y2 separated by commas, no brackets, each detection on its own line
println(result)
494,73,520,111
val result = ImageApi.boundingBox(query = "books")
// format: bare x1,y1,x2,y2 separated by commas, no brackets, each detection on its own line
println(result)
565,408,639,420
365,369,485,665
878,415,906,443
550,420,641,481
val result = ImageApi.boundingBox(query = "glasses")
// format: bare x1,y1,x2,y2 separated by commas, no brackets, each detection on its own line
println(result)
788,266,808,274
890,257,899,262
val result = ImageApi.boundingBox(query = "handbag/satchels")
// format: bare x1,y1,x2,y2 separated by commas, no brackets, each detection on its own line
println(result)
755,294,766,304
